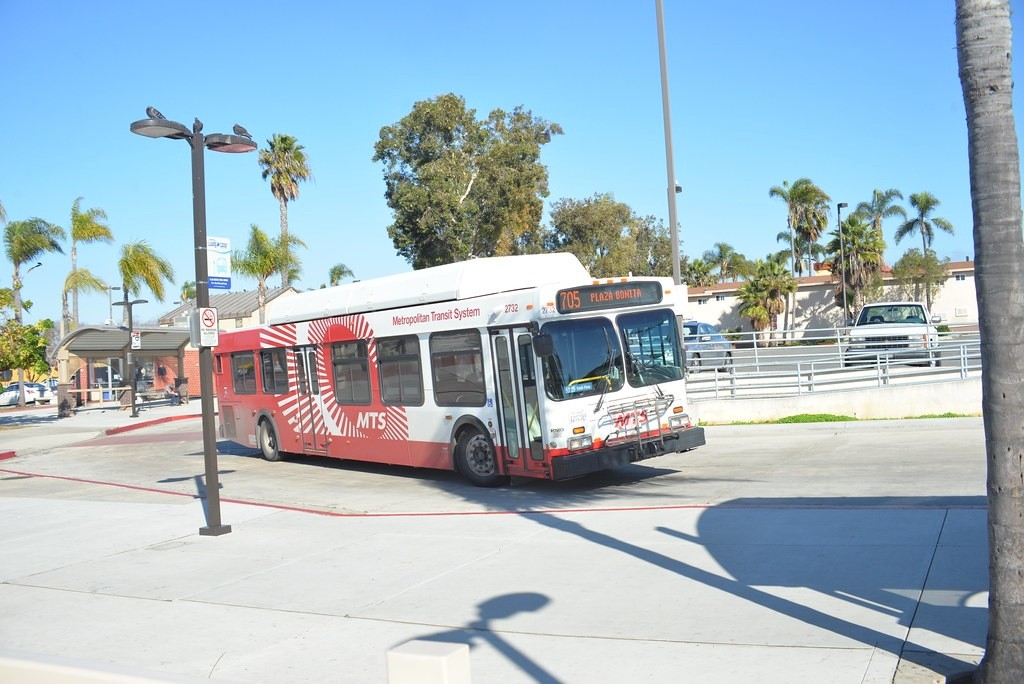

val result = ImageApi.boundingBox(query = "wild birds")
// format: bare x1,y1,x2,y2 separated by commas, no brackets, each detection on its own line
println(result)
145,105,168,121
232,123,251,139
193,115,203,133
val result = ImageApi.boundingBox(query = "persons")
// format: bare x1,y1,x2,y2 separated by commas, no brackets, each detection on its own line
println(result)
164,383,180,407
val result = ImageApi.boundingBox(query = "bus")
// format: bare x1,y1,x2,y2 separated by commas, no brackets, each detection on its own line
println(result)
213,253,706,487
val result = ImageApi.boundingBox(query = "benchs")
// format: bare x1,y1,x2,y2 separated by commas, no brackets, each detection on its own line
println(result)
133,391,181,409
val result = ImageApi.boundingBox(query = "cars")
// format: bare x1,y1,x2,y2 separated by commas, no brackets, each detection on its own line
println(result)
0,379,59,408
844,302,941,367
630,321,734,373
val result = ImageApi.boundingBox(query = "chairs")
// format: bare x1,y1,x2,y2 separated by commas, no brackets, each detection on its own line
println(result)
906,316,919,324
870,316,884,323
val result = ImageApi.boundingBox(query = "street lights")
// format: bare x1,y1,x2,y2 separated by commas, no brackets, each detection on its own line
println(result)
112,300,148,417
101,286,121,319
837,203,848,335
130,106,258,536
